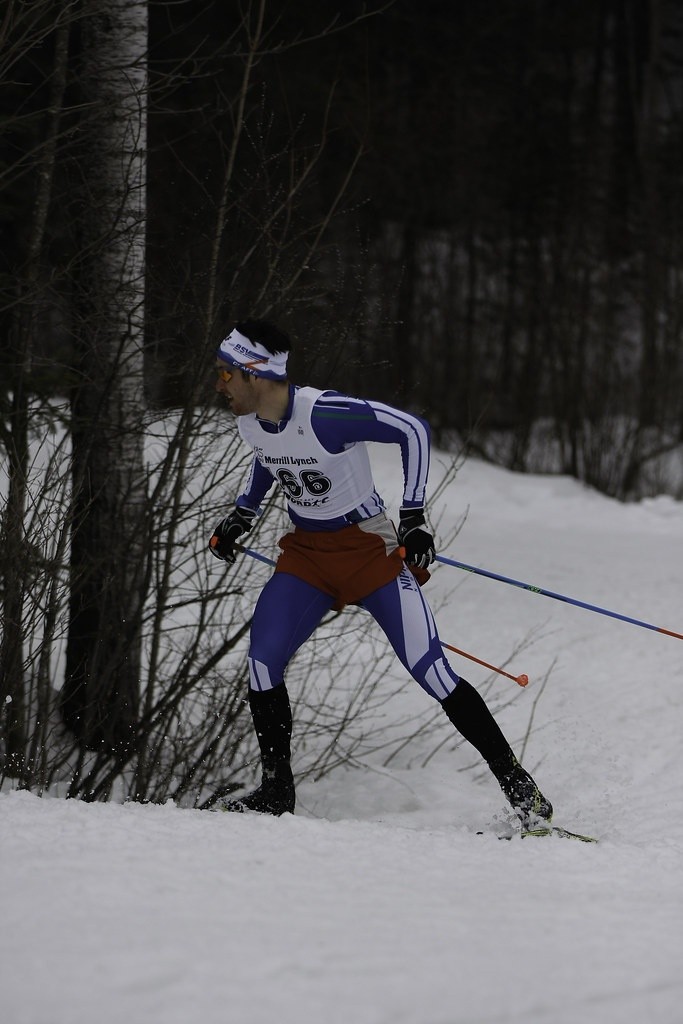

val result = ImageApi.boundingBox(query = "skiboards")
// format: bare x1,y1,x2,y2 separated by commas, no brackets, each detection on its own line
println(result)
493,811,595,844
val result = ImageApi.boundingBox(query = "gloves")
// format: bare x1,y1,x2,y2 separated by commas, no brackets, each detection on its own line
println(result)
397,506,435,570
208,505,256,566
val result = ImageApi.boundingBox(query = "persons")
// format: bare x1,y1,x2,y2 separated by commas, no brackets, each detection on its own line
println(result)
205,319,554,835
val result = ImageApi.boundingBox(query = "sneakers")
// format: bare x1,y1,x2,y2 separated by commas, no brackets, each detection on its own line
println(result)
488,750,553,835
224,780,295,816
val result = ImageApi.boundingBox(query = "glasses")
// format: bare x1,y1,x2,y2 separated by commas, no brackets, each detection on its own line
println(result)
213,359,268,383
